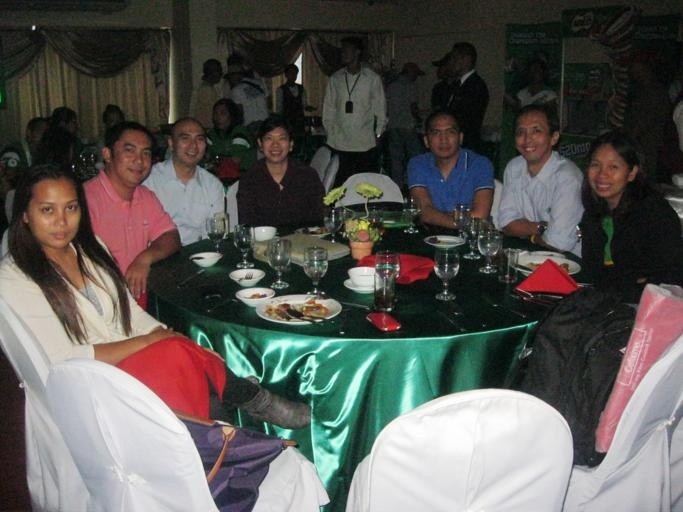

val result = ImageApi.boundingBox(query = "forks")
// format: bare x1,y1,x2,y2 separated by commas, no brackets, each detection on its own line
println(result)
274,308,317,327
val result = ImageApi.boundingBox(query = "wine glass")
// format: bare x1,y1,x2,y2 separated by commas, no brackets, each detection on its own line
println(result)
325,208,343,243
375,249,459,301
205,218,225,255
234,224,254,269
402,197,423,235
454,205,504,274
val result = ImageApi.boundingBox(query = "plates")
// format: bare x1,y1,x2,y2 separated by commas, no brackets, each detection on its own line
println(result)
259,293,342,324
423,235,464,250
343,280,381,294
514,251,583,276
294,227,331,239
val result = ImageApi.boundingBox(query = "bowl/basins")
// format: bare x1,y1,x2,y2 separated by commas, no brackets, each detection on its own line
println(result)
230,269,275,307
189,252,222,268
250,226,277,242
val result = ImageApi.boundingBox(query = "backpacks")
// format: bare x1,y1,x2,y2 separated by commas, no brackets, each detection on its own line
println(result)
508,287,637,468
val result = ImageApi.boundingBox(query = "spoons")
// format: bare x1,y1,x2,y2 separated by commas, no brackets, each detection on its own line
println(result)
288,310,335,326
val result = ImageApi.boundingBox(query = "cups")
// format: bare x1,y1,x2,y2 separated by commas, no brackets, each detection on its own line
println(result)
265,240,292,288
375,266,397,313
215,212,228,239
303,247,328,296
348,267,376,288
340,207,386,236
497,249,518,283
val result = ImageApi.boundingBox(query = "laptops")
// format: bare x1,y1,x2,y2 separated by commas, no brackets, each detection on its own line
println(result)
253,233,350,266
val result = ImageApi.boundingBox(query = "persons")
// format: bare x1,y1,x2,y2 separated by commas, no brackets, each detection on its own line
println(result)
1,34,683,430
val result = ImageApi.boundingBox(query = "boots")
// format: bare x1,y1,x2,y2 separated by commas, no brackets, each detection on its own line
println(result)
238,376,311,429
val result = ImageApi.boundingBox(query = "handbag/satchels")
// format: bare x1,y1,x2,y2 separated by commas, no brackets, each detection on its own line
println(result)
172,410,298,512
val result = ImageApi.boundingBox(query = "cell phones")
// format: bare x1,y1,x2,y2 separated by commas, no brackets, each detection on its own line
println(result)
366,313,401,332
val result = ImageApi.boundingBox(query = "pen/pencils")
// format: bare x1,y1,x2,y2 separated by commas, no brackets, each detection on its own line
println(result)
177,268,204,289
